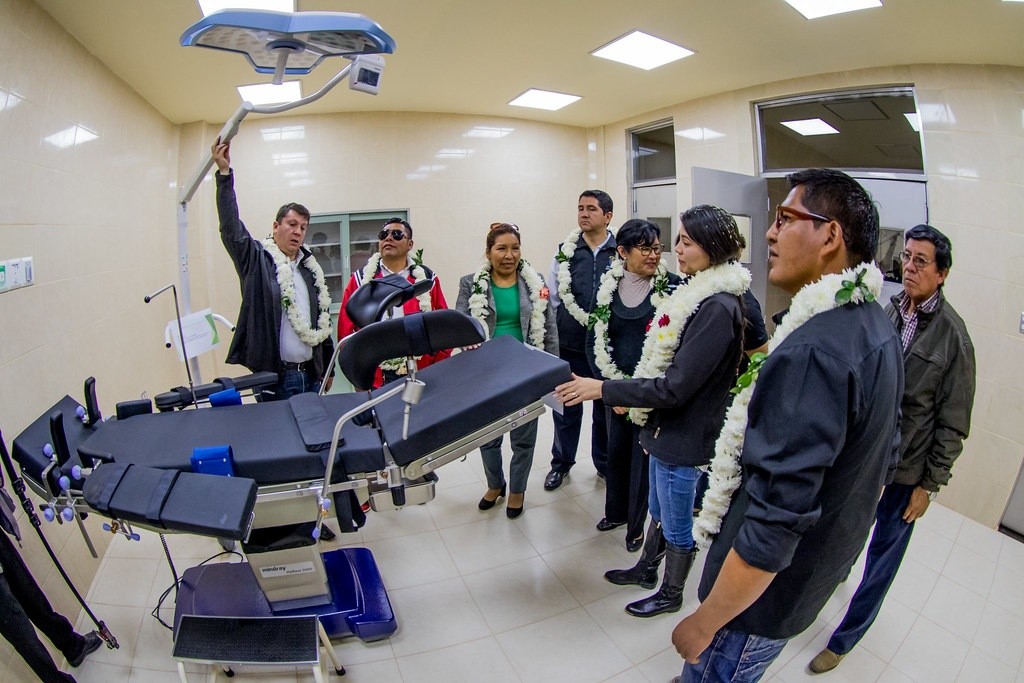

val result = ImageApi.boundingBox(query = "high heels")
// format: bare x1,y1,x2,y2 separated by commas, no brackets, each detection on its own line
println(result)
479,480,506,510
507,493,524,519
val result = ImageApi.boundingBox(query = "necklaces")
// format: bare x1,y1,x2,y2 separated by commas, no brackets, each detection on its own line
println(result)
630,261,753,426
468,258,550,352
588,259,668,380
691,260,884,549
556,226,619,326
361,249,432,370
261,234,332,347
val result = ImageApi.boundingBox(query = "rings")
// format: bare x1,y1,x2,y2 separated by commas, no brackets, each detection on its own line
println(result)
573,391,577,398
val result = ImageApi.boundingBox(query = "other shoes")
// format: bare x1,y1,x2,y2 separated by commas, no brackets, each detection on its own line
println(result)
810,647,846,673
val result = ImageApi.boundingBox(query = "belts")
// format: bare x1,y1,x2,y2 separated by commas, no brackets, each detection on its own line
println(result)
281,362,308,369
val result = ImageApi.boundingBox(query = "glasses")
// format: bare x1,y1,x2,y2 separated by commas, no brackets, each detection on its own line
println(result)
378,230,409,240
490,223,519,231
898,251,935,268
775,205,832,229
634,243,665,257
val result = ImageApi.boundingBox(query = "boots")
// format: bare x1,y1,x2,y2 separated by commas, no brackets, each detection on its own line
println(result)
626,542,697,618
604,518,667,590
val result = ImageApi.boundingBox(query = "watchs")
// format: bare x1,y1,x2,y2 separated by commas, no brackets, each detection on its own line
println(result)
924,489,937,501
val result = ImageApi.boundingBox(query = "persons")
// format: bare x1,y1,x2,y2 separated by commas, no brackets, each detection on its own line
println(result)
810,225,975,672
456,223,560,520
310,233,328,272
337,217,454,514
349,236,371,271
545,190,617,491
672,168,905,683
554,203,768,618
210,134,336,541
585,219,682,552
0,462,102,683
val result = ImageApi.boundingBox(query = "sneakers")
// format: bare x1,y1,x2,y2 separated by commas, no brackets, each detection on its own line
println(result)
359,500,370,511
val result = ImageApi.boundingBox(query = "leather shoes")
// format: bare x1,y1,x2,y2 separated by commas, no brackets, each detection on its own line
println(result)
69,631,103,667
544,468,569,492
596,518,626,531
626,527,644,552
